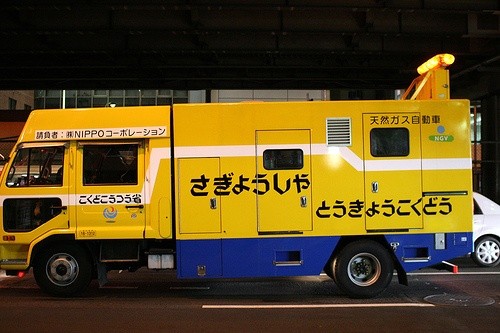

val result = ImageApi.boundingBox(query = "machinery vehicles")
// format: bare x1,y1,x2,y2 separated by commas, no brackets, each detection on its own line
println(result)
0,54,473,294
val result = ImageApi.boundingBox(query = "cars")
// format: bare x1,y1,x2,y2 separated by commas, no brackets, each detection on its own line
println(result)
472,192,500,266
